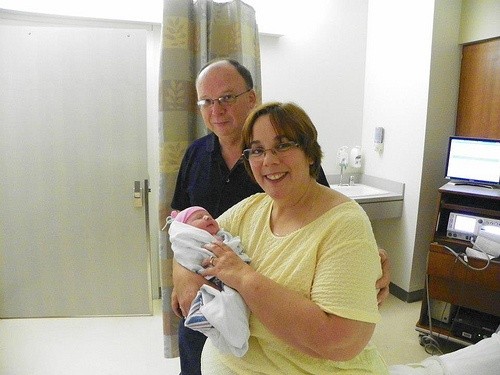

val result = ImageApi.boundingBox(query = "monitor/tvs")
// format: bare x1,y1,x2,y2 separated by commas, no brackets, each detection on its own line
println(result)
443,136,500,188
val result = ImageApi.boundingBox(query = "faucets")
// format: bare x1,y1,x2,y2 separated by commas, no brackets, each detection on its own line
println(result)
337,158,356,186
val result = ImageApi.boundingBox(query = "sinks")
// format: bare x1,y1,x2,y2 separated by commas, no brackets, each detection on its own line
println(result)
329,184,389,197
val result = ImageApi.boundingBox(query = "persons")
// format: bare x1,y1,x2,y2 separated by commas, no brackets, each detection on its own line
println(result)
165,206,250,358
170,58,389,375
173,103,384,375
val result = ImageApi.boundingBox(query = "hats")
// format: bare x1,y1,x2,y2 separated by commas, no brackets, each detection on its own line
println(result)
171,206,210,224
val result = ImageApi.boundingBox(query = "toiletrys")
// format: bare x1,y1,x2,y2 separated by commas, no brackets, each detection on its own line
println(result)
351,146,361,168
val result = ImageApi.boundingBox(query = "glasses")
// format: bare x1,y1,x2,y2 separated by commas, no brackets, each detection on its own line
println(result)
197,88,251,108
241,142,299,162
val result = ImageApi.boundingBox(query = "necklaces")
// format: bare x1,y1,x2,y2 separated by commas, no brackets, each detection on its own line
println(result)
270,184,322,237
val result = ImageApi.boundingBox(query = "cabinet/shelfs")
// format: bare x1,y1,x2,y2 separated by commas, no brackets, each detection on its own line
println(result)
414,197,500,347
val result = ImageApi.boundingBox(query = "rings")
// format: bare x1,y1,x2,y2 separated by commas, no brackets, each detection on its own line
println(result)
210,256,214,266
